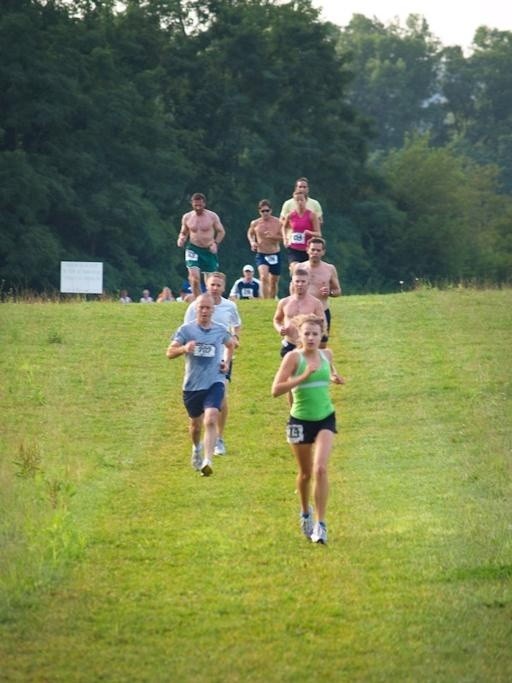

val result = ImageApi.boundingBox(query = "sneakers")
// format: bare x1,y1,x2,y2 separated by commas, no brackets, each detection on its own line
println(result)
192,445,202,469
301,506,313,537
312,521,326,544
201,459,213,476
215,437,225,454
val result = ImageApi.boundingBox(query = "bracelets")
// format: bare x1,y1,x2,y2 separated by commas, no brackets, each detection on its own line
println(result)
232,334,239,339
214,240,219,244
329,289,335,296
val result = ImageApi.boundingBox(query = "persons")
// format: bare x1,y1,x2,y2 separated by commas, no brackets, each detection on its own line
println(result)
277,177,324,238
271,267,328,409
175,191,226,300
282,191,321,296
182,269,243,456
119,262,263,302
247,198,284,300
270,314,348,544
166,292,236,478
288,235,342,349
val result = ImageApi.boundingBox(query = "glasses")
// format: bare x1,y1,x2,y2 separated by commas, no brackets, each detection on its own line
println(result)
261,209,269,212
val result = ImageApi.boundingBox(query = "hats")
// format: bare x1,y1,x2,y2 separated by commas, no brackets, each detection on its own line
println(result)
243,265,253,271
